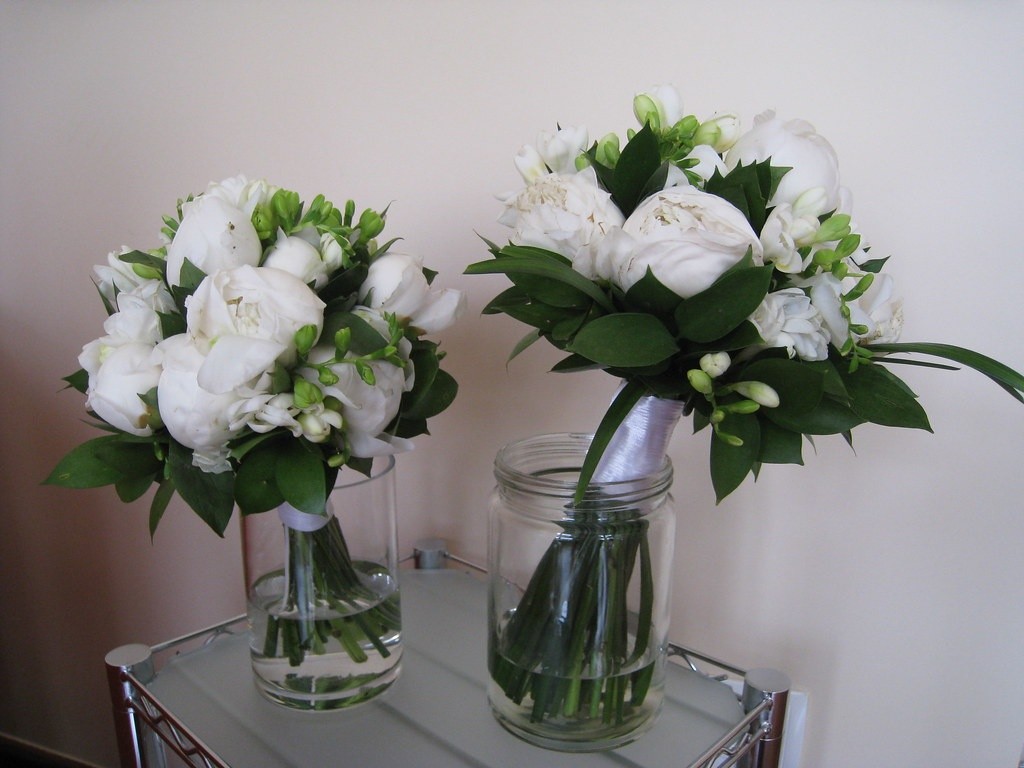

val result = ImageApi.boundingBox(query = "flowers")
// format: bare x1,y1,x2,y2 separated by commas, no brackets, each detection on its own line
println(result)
38,176,461,667
460,94,1024,731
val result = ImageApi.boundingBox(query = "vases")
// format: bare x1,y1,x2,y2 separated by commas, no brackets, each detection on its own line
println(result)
239,452,403,714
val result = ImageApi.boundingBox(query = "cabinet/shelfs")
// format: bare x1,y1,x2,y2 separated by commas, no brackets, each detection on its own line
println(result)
105,539,789,768
